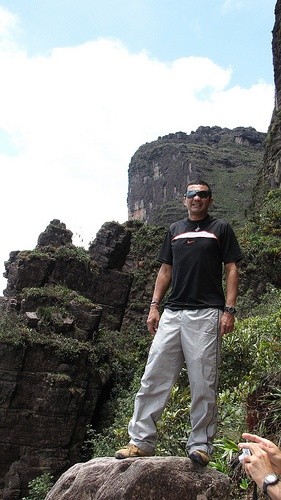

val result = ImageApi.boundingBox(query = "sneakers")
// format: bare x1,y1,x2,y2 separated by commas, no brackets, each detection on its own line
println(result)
115,443,151,459
188,450,210,467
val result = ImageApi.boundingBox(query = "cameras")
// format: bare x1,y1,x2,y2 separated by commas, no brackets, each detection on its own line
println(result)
239,447,252,463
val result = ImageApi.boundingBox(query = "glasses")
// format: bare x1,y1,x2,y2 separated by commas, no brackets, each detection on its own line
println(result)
187,190,209,199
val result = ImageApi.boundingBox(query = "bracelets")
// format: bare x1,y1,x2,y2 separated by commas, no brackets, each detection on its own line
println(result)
150,302,159,308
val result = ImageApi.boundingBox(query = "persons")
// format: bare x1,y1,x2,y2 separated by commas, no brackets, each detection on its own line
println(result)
115,179,242,467
237,432,281,500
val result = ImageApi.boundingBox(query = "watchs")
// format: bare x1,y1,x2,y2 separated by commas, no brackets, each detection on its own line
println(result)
224,306,237,315
262,473,280,497
